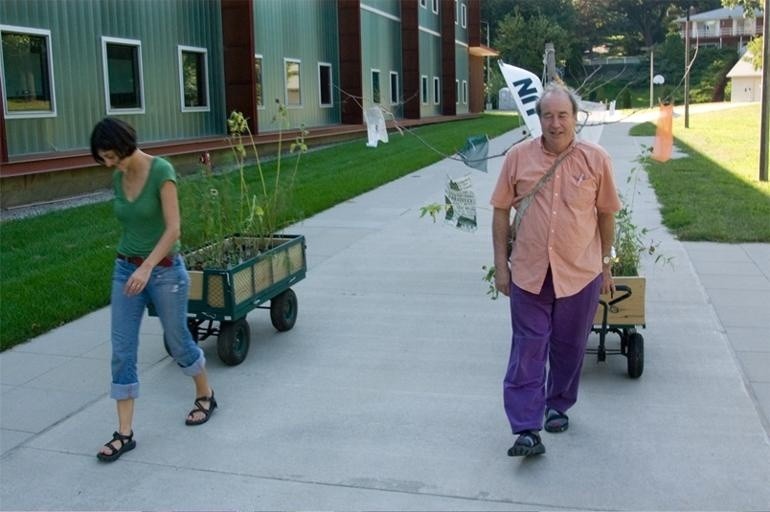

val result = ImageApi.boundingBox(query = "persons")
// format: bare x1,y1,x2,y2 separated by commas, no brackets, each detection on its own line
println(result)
90,117,217,463
490,83,623,456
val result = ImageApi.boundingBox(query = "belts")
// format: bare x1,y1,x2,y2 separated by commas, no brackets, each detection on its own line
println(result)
117,253,173,266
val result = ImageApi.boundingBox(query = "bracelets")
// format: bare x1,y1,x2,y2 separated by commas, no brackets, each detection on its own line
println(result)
602,256,611,265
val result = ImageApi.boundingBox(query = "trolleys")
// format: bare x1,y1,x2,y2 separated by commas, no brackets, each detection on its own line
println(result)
584,251,646,378
147,232,306,366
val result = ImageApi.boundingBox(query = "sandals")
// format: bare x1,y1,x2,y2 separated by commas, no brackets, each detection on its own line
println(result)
97,431,136,461
545,408,568,432
508,431,545,456
186,390,217,425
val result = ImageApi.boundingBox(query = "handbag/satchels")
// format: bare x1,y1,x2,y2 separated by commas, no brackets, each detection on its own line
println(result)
507,225,516,260
